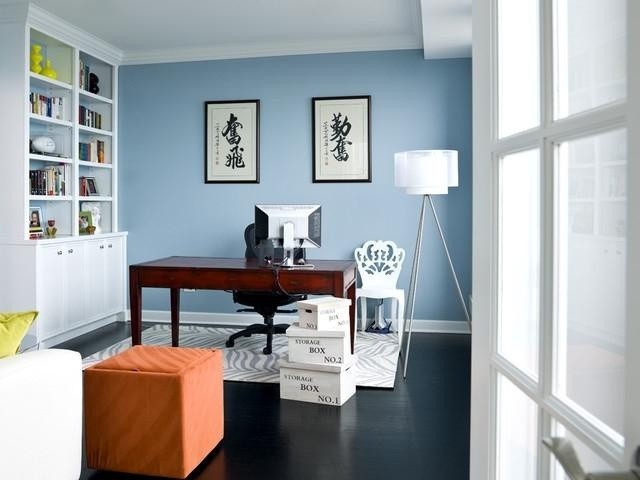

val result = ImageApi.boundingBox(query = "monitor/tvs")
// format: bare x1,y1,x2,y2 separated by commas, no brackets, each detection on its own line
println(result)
255,203,322,267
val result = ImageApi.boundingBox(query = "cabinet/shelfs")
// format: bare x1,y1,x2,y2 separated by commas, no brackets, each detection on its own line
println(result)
77,232,128,331
1,3,125,238
0,240,77,345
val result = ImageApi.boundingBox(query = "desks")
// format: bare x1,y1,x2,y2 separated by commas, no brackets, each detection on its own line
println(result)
125,254,358,355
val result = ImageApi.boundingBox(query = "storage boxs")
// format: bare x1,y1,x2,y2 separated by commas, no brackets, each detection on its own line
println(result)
276,350,357,407
285,320,351,366
295,293,351,330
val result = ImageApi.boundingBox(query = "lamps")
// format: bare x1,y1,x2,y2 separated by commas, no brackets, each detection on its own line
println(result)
387,146,471,381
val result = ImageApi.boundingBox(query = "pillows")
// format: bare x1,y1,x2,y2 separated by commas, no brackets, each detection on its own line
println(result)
0,308,41,360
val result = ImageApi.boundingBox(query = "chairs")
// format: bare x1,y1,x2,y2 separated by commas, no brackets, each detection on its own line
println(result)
349,238,411,350
0,345,85,480
221,221,312,357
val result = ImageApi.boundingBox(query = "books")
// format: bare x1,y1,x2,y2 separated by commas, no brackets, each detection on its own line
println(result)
30,88,102,130
79,140,104,164
30,164,72,194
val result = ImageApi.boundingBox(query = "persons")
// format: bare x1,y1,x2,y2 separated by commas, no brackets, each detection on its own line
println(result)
80,217,89,228
30,212,39,226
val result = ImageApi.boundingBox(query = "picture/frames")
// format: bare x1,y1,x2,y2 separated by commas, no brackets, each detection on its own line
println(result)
308,93,374,187
202,95,263,186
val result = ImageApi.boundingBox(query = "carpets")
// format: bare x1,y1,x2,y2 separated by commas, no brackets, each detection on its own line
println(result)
78,322,406,391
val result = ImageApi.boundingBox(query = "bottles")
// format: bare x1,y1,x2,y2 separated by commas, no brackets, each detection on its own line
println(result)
30,44,44,74
39,60,57,80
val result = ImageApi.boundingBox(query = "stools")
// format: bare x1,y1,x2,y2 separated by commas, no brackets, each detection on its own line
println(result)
82,342,227,480
